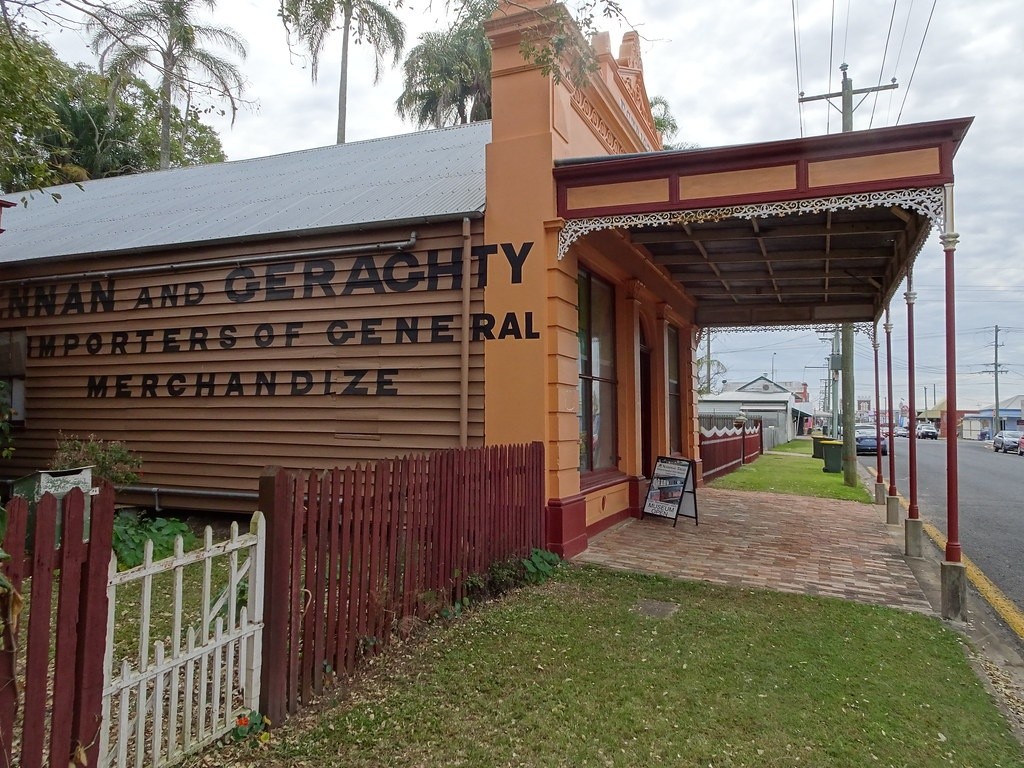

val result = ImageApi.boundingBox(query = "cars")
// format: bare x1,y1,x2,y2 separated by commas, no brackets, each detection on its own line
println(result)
993,430,1024,453
1017,434,1024,456
838,420,938,456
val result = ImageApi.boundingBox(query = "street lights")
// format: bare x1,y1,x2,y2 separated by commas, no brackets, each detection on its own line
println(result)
923,386,930,423
771,351,777,382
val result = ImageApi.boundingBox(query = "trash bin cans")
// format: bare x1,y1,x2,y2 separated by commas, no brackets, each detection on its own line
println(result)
819,440,844,473
823,426,828,436
811,436,832,459
